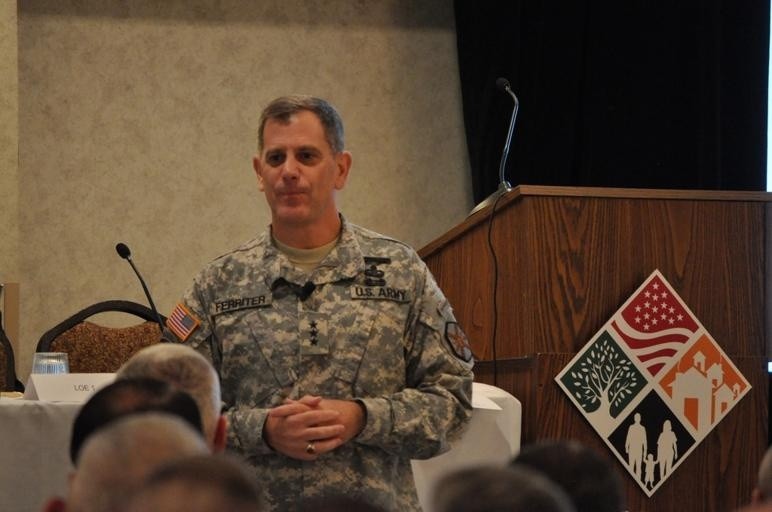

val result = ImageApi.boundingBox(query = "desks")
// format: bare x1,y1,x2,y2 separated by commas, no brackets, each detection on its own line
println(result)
0,398,82,512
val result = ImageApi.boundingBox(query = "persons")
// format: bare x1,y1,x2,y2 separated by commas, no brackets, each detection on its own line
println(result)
748,444,772,512
153,95,473,512
37,343,628,512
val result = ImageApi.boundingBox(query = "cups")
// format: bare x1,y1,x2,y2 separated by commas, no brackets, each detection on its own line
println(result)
32,352,70,400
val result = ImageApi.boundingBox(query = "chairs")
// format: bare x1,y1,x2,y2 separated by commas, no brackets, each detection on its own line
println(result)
36,300,166,373
0,328,16,392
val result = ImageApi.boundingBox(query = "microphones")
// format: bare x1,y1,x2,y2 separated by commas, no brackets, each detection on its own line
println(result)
114,240,166,334
497,76,520,197
